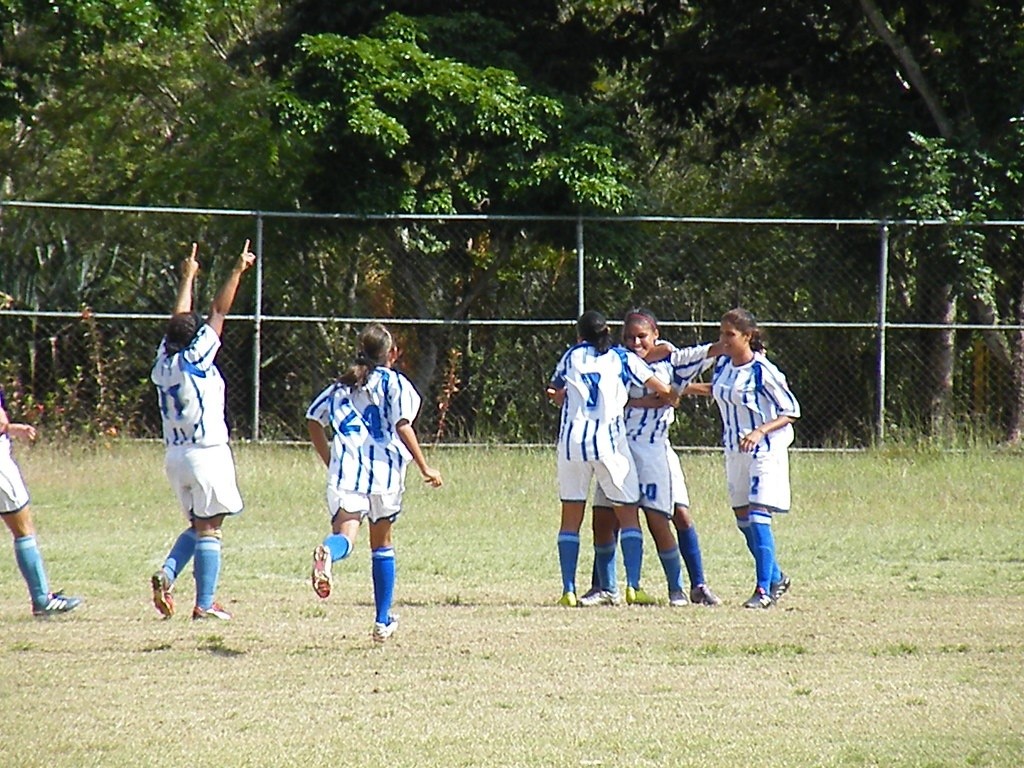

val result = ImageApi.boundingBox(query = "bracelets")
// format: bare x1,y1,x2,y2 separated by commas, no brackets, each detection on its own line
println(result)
757,429,766,434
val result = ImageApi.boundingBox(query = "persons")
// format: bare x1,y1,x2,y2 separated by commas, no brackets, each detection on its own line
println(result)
0,403,82,617
304,322,444,643
150,237,258,621
550,308,680,610
681,306,802,611
575,308,727,607
575,321,726,609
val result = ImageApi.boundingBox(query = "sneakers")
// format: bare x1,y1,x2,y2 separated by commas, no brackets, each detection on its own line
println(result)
669,590,689,607
312,545,332,599
578,590,620,607
373,616,400,642
626,587,655,604
152,571,174,616
690,584,722,606
557,592,576,607
771,573,791,601
745,591,776,609
582,589,599,597
193,603,233,622
32,593,81,616
